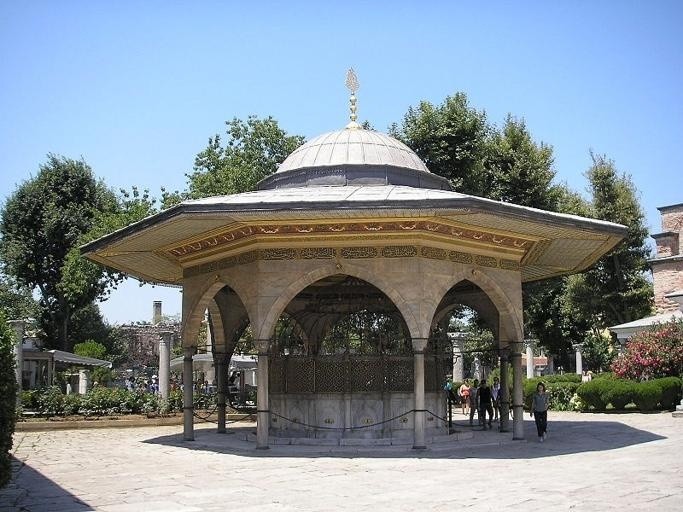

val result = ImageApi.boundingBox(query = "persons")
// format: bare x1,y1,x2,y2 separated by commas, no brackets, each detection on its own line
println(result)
125,376,211,395
444,380,452,391
582,368,593,376
530,382,549,442
459,377,500,427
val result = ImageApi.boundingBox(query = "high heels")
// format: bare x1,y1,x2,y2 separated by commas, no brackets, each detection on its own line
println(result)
540,432,547,442
